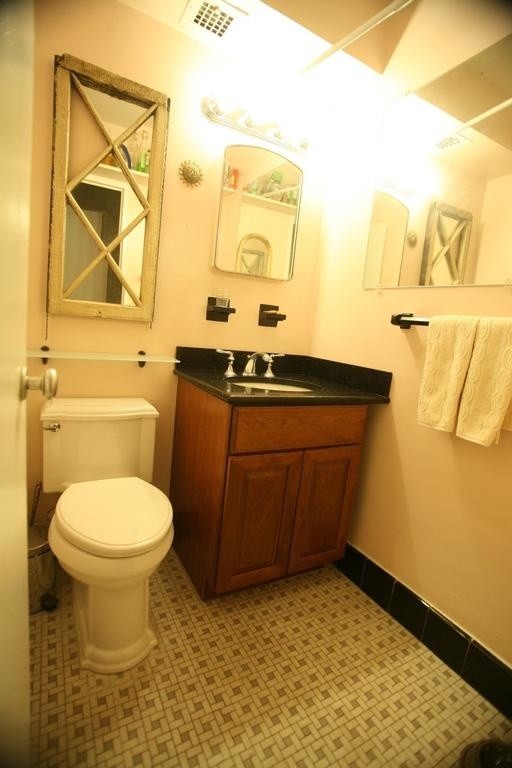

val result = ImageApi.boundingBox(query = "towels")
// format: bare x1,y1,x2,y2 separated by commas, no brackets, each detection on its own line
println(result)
454,316,512,448
421,308,484,434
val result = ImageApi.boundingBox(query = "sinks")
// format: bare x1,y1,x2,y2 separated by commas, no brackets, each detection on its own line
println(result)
224,377,323,393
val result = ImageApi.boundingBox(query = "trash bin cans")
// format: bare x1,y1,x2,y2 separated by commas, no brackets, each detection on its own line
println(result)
27,525,60,615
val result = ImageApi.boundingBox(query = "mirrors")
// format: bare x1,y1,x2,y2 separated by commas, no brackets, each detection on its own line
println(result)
363,188,410,289
61,75,158,306
422,199,473,286
214,141,306,283
363,31,511,290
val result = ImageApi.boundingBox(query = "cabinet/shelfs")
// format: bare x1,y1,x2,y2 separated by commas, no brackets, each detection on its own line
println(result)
172,379,369,601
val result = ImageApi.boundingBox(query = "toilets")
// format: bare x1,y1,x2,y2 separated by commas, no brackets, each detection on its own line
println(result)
40,396,175,675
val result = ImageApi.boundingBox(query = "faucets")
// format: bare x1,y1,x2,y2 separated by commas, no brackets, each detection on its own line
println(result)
242,352,262,376
